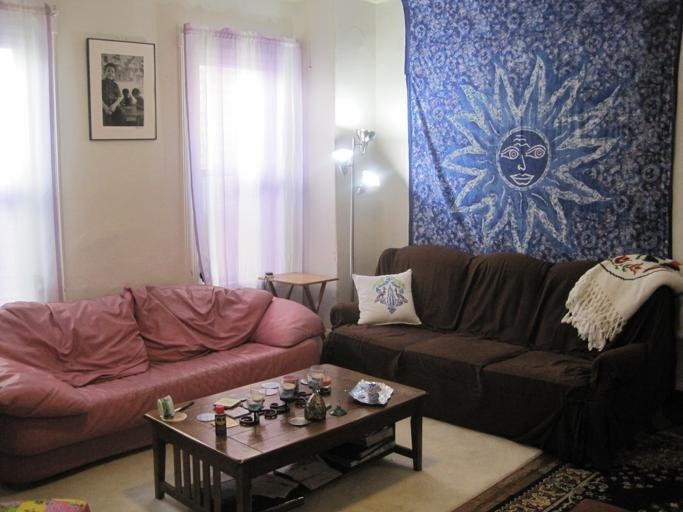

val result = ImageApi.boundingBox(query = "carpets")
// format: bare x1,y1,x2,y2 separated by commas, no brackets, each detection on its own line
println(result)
0,410,545,512
449,432,682,511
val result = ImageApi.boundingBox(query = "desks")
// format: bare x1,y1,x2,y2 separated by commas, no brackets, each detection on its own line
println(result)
257,271,339,314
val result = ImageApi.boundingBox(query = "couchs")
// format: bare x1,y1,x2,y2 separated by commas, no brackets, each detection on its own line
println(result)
0,281,326,489
328,242,681,468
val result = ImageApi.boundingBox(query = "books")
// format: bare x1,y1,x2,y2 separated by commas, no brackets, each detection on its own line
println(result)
275,421,395,489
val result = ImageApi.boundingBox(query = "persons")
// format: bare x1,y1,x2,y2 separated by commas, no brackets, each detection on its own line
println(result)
101,64,144,126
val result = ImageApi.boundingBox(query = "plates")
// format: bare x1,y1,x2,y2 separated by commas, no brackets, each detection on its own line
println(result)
161,412,187,422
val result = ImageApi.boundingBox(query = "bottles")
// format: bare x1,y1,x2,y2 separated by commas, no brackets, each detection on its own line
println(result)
215,435,227,452
214,405,227,435
305,386,326,422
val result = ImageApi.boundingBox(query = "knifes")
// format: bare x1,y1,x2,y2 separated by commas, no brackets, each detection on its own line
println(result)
176,400,194,412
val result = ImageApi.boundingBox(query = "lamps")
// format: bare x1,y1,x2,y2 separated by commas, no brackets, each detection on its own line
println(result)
331,127,379,301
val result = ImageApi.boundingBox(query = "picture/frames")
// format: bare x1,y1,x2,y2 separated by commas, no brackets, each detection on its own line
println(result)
86,37,158,142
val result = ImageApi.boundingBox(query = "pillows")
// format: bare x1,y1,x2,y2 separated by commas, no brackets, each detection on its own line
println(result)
351,268,424,325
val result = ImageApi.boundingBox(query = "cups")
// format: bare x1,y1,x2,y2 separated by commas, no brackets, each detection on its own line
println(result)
247,385,267,410
279,374,300,398
157,394,175,418
307,363,331,394
